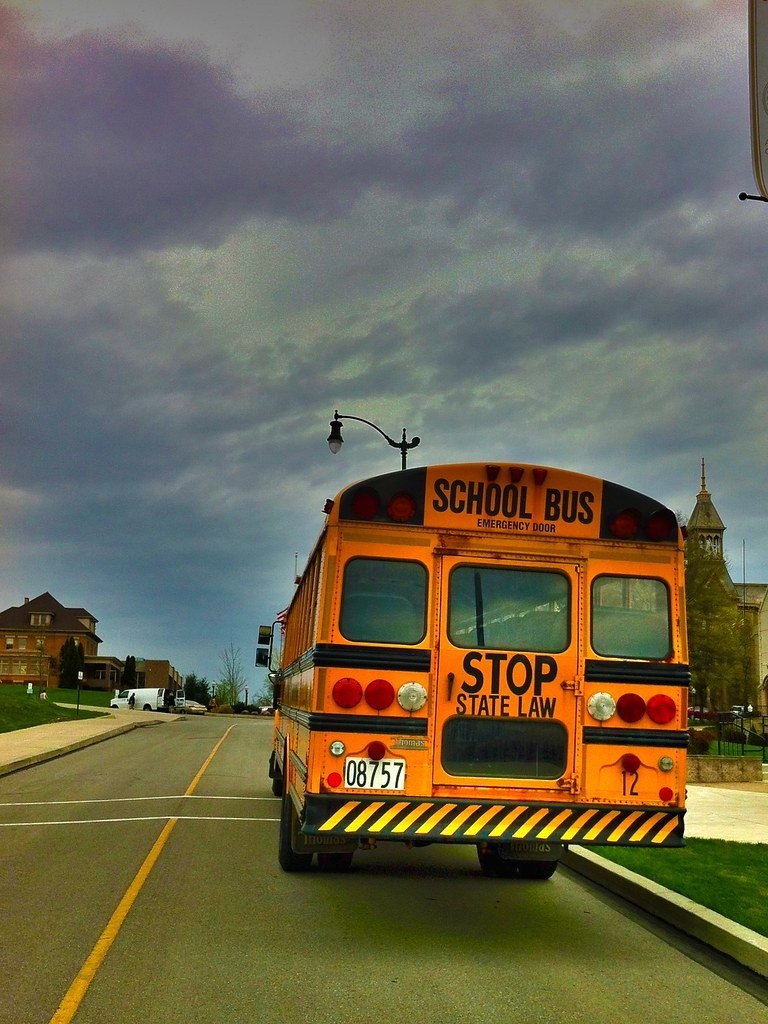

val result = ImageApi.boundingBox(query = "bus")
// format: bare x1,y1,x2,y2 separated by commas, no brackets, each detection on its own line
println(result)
253,459,692,880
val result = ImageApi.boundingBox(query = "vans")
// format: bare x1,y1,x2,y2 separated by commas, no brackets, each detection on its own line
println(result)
110,688,186,712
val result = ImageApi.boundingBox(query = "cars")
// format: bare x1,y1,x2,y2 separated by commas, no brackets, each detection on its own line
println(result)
169,700,207,715
729,705,745,717
687,705,720,720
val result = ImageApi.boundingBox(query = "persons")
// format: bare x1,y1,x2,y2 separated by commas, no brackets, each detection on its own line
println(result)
128,693,136,711
40,690,48,700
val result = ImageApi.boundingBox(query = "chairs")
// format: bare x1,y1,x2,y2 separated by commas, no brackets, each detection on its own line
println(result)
339,591,670,659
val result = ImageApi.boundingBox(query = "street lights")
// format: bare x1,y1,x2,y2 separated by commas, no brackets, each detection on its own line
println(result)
212,680,217,696
328,407,421,473
244,684,249,708
